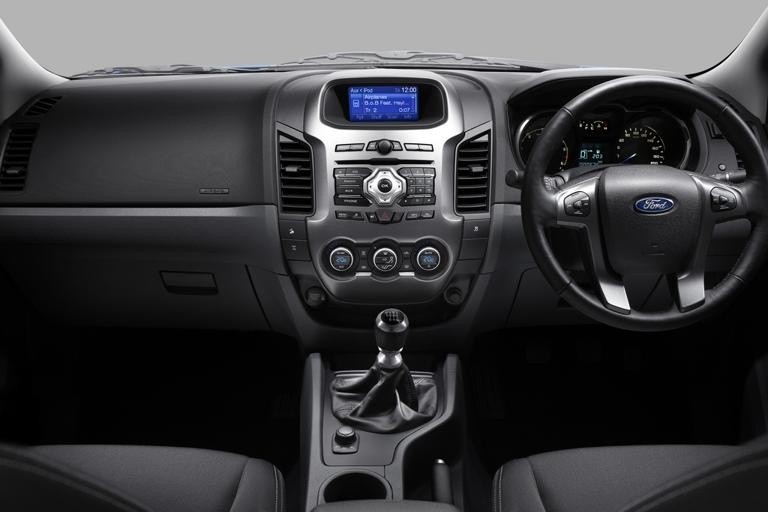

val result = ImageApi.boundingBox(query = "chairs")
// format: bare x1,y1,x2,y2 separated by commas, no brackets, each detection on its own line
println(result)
490,430,768,511
0,444,285,512
